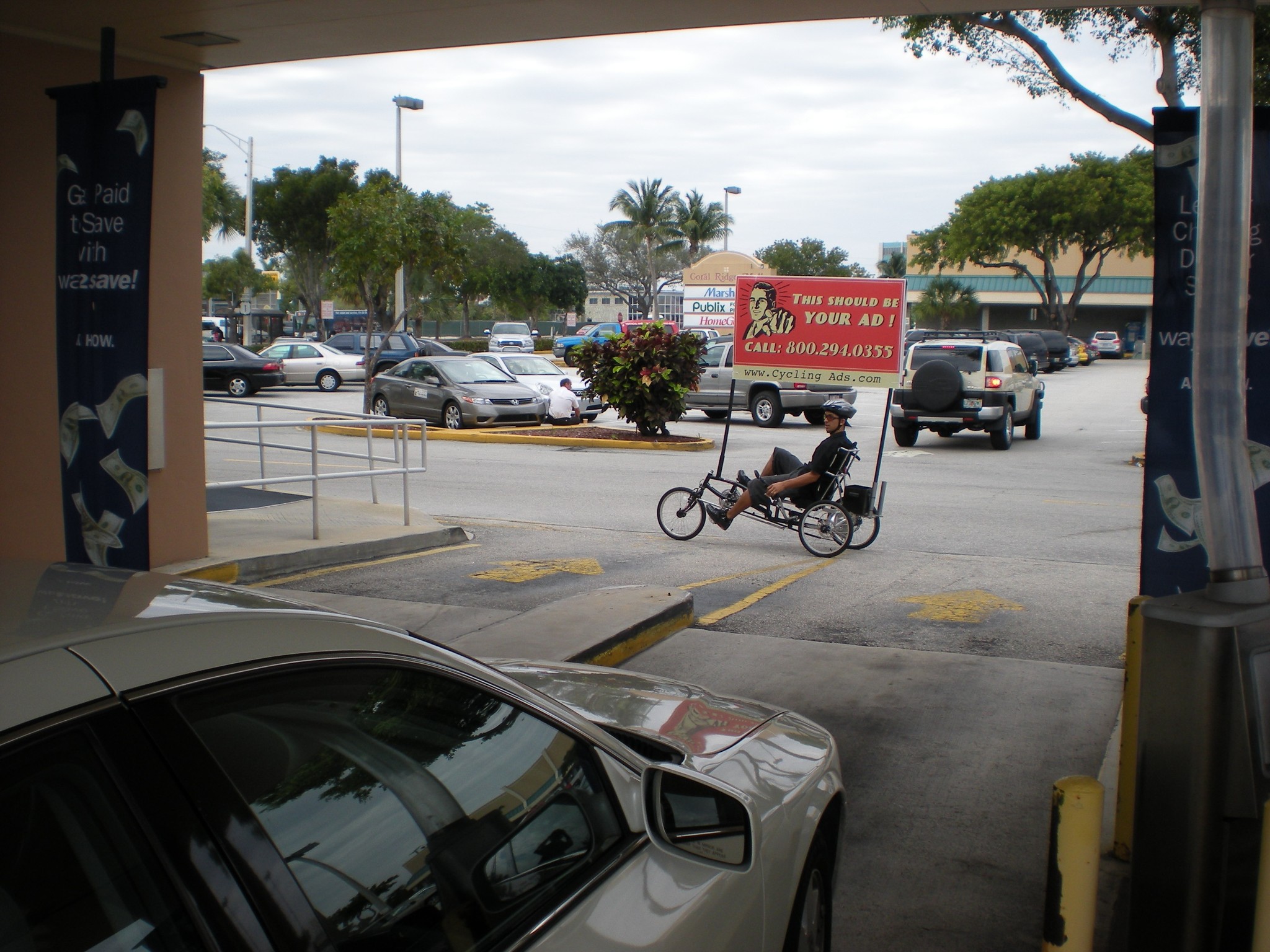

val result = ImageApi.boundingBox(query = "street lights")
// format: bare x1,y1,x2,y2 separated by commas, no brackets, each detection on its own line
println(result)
723,186,742,251
203,123,254,347
392,94,424,335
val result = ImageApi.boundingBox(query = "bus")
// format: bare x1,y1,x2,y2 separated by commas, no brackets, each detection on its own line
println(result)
294,308,370,337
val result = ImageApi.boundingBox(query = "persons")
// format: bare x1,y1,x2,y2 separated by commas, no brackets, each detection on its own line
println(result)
705,398,857,531
237,326,243,346
210,326,223,342
547,379,580,426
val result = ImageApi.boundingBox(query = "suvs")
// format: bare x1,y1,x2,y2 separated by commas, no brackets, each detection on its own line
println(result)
887,329,1046,451
902,327,1103,377
322,332,434,379
1090,331,1124,360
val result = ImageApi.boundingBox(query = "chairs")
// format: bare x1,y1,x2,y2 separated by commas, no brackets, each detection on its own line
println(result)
513,365,523,373
419,367,432,380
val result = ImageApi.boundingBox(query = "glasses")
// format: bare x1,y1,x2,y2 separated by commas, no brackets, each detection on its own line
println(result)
824,413,844,421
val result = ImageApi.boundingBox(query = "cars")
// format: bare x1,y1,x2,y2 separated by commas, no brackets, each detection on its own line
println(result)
271,336,309,351
415,338,472,357
484,321,539,354
255,340,372,392
550,319,859,429
465,352,606,423
371,354,552,429
202,341,287,398
202,316,269,346
0,550,846,952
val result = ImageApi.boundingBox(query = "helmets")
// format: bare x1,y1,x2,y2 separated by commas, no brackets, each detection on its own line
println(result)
821,398,858,418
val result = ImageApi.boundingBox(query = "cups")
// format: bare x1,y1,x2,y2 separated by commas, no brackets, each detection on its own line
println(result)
583,419,588,424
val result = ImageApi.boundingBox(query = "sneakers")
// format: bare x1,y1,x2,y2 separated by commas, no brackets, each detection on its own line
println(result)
738,470,751,491
705,503,734,531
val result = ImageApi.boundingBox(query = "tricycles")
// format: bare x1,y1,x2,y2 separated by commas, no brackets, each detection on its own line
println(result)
654,445,883,559
